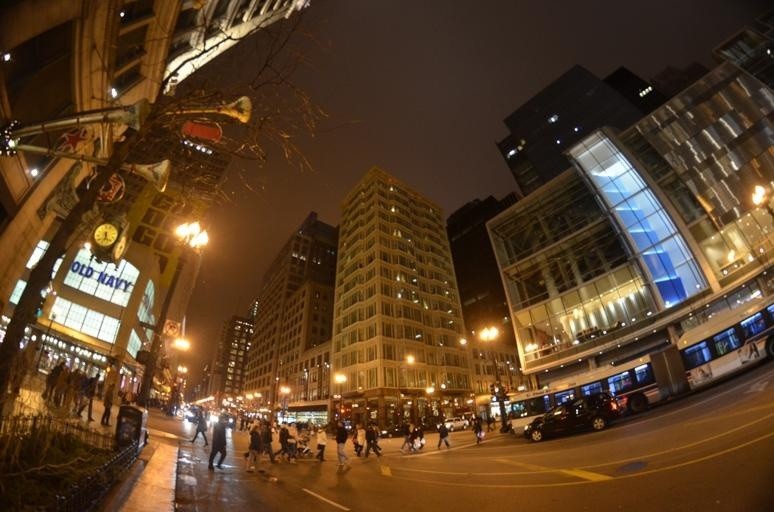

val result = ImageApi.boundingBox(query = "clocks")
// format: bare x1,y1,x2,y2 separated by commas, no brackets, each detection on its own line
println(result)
91,214,130,265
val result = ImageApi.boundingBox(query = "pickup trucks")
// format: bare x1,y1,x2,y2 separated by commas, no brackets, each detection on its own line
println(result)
436,417,470,433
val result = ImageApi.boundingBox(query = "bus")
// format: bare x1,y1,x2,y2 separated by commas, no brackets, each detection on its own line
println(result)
506,294,774,437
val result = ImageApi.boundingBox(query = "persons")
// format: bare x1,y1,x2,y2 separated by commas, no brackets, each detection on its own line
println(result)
400,424,412,454
191,409,209,444
412,426,420,451
209,413,230,469
239,417,279,432
41,360,100,422
243,419,383,473
472,418,483,444
487,415,493,432
438,421,450,449
492,416,496,429
418,425,424,449
118,390,170,410
101,383,116,426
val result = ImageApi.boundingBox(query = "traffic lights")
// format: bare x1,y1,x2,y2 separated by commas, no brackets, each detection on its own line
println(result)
487,379,510,403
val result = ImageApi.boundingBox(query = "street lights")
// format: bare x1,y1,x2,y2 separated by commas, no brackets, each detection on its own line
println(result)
227,391,263,427
335,373,347,421
165,334,191,417
478,322,513,435
280,385,291,425
131,218,211,446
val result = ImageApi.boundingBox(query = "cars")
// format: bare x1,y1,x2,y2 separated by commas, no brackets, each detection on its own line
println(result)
376,421,420,438
342,420,356,434
523,389,620,441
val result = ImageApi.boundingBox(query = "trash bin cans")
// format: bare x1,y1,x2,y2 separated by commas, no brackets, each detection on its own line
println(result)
115,405,149,452
